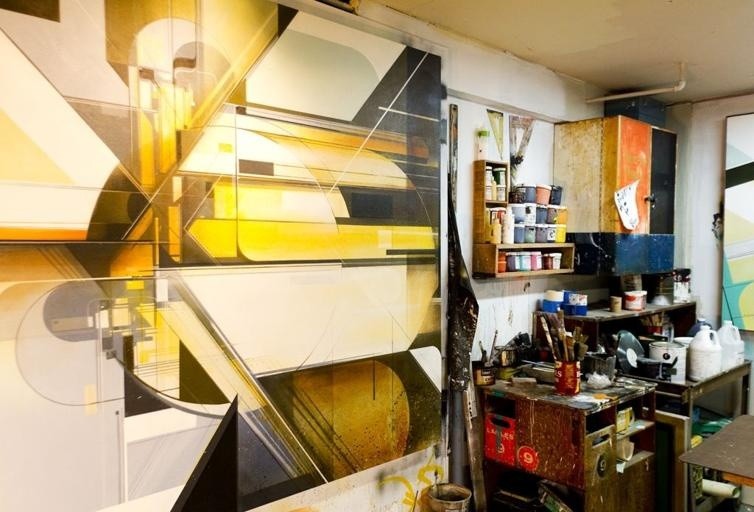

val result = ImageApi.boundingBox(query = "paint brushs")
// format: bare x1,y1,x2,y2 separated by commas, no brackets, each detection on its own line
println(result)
539,310,588,361
479,330,522,368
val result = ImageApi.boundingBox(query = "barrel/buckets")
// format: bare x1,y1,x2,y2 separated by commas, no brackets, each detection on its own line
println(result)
689,326,722,381
427,482,471,512
687,317,714,338
717,319,745,371
649,322,687,384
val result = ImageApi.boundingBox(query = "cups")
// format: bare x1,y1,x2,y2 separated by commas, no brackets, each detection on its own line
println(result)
610,296,622,312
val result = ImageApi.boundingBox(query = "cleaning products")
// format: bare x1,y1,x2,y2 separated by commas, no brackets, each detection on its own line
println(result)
500,205,514,244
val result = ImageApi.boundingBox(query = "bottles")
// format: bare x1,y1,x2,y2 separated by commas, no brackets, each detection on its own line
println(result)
486,206,514,243
478,132,490,160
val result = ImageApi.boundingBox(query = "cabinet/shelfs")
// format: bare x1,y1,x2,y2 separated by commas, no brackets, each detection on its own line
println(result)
476,161,577,277
481,302,752,512
606,115,678,276
